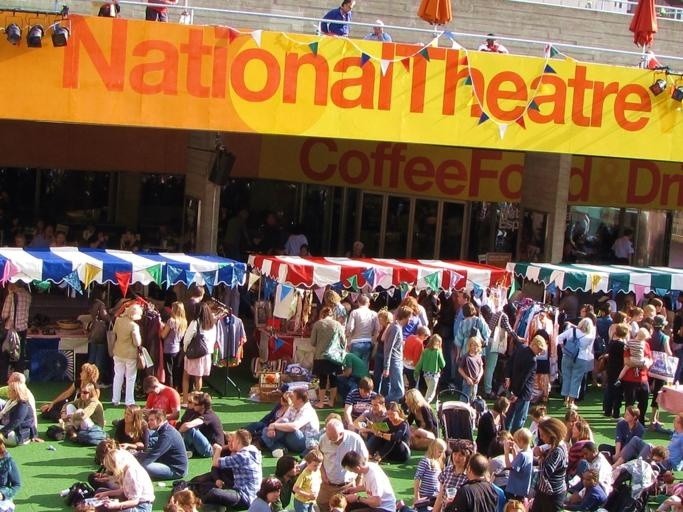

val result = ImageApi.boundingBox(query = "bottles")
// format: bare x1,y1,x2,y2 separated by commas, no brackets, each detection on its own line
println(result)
675,380,680,390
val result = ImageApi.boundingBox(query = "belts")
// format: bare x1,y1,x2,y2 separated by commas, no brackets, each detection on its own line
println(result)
328,480,351,487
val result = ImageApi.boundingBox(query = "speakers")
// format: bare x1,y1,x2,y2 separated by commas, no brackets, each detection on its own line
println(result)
209,153,236,186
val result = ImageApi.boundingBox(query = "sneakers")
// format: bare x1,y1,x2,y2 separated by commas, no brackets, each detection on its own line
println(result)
613,380,622,387
563,401,579,410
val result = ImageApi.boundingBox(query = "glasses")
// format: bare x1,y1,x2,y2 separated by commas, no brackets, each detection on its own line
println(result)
80,390,90,394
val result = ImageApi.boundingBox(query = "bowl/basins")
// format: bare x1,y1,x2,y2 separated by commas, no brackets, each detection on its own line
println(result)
57,319,83,329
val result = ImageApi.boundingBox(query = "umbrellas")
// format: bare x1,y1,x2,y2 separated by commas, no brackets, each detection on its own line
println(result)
417,0,452,34
629,0,657,69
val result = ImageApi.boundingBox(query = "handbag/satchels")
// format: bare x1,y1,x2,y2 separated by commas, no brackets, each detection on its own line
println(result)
595,353,610,373
593,321,610,352
104,319,119,358
184,318,210,360
534,358,551,375
489,311,510,355
90,302,108,344
467,316,489,349
645,335,681,385
1,327,22,354
133,342,154,370
322,319,349,366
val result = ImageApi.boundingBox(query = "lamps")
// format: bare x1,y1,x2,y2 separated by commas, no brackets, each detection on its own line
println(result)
4,9,71,47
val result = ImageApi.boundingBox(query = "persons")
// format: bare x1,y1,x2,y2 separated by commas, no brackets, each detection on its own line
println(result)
563,221,635,265
97,0,121,17
0,188,197,252
478,33,509,54
0,285,681,512
218,209,542,261
145,0,178,22
363,19,392,42
320,0,355,37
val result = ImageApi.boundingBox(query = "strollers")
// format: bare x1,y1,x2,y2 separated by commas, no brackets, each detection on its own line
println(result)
588,456,662,512
434,386,479,458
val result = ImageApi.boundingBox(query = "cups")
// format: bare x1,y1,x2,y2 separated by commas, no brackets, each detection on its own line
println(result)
444,487,458,498
667,381,672,387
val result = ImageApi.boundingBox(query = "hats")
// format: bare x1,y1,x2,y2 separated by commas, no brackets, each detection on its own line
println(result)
652,314,669,327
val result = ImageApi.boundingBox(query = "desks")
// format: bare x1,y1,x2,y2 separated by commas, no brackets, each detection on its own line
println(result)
26,322,88,382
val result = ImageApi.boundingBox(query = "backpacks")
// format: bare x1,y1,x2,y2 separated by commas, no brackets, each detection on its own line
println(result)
560,326,587,362
